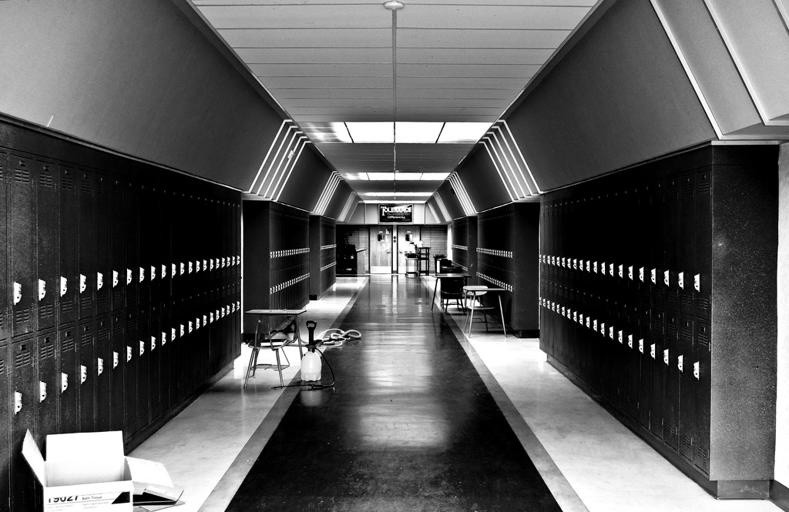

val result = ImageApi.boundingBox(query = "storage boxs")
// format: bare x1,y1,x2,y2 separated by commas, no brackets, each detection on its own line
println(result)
21,426,185,512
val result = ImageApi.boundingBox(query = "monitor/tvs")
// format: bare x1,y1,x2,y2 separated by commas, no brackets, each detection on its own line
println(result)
377,203,414,225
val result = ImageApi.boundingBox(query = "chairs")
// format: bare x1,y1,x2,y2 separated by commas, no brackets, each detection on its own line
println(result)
243,317,290,388
440,265,495,332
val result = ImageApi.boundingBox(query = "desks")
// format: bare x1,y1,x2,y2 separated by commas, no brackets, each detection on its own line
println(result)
244,309,307,376
431,272,508,339
